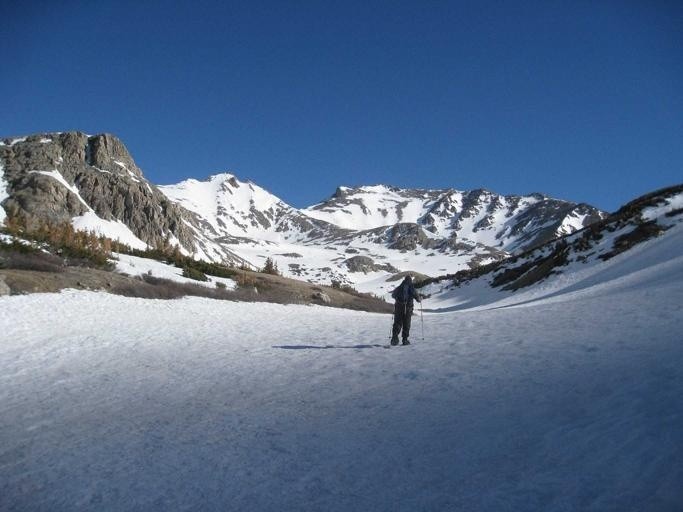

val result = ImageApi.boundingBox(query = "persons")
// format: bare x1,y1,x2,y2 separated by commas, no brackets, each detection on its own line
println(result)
390,275,422,345
